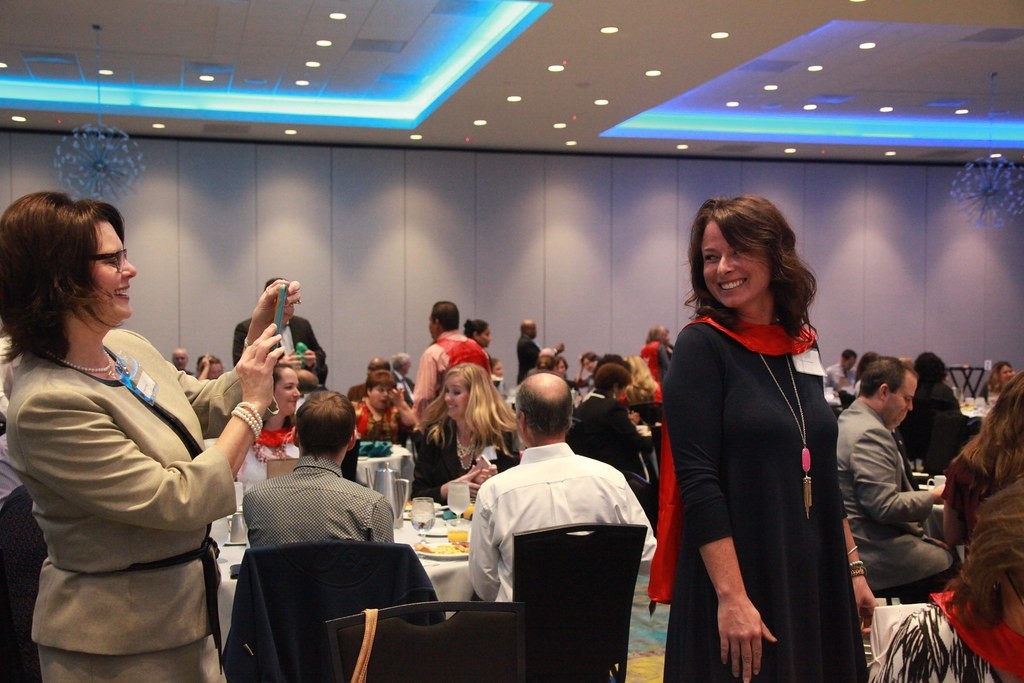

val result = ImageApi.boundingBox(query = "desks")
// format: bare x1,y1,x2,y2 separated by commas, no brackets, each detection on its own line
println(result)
961,399,991,419
204,437,415,546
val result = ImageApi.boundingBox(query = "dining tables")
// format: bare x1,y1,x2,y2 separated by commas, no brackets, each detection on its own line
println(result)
218,495,474,651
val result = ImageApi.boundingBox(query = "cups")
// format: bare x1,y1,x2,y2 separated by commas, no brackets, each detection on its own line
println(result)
233,481,244,511
227,510,247,542
928,475,946,490
965,397,973,405
447,530,468,543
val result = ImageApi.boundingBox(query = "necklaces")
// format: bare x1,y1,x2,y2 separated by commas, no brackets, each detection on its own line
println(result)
758,353,813,519
455,432,476,469
38,343,112,372
252,439,287,463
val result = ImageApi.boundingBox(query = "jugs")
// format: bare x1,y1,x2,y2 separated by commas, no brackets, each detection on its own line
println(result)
364,462,410,529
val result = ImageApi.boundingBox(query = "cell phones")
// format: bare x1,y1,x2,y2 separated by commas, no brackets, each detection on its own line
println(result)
271,284,288,351
471,455,491,485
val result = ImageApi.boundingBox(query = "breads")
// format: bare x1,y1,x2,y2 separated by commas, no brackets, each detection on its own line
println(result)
414,543,433,553
452,542,469,553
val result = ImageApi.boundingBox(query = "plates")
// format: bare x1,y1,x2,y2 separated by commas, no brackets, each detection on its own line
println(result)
419,531,447,536
402,510,443,520
413,543,468,561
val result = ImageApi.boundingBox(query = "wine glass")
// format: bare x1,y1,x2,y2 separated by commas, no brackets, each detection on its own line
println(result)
448,482,471,526
411,497,434,546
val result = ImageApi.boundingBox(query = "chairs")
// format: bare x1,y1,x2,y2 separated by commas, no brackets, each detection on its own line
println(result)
511,522,647,683
325,601,524,683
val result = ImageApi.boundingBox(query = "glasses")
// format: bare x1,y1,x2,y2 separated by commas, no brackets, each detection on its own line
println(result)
91,250,128,272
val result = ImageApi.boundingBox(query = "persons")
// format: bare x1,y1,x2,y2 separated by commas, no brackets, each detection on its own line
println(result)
645,190,878,683
823,350,1024,477
517,318,565,385
469,372,657,602
867,368,1024,683
575,352,601,388
347,357,391,403
525,356,566,381
350,369,416,447
490,358,504,398
0,191,328,683
836,356,963,604
242,391,394,548
410,301,492,455
411,363,520,506
555,357,568,375
565,326,675,537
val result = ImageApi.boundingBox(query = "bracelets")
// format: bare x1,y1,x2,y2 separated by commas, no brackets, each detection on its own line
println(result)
231,402,263,441
847,546,864,577
